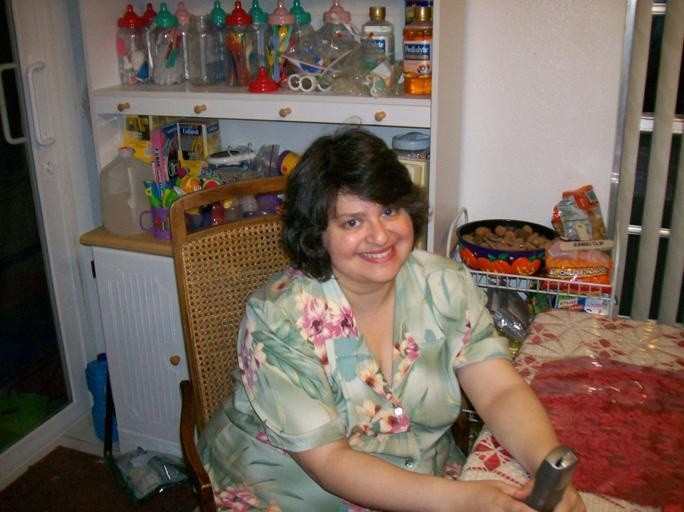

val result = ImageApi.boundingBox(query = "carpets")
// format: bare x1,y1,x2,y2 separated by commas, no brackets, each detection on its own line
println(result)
0,445,201,512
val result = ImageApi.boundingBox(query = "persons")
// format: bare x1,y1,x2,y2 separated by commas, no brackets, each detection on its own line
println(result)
194,127,587,512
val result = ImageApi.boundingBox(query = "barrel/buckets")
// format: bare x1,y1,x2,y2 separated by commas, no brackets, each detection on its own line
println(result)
99,147,152,236
86,354,118,441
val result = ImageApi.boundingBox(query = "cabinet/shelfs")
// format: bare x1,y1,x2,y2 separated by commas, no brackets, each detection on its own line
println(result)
78,0,621,476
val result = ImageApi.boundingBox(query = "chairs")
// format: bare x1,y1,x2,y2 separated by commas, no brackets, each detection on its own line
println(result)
168,171,298,512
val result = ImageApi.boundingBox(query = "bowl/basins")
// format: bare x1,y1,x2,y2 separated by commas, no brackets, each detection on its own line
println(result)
456,219,560,277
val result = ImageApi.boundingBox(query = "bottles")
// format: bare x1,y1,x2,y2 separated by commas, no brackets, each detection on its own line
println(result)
116,1,432,96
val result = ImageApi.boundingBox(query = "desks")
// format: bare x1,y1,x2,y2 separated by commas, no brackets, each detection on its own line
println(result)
454,308,684,512
